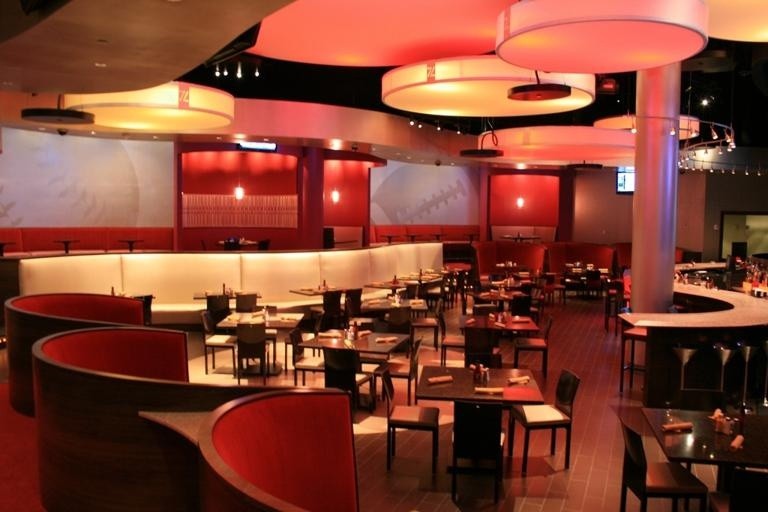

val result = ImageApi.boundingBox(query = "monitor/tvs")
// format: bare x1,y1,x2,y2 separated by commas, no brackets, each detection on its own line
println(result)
615,166,634,195
236,142,278,151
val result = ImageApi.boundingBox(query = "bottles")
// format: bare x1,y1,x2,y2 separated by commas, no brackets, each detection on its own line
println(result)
723,416,741,437
348,320,358,341
664,400,674,424
472,363,490,386
